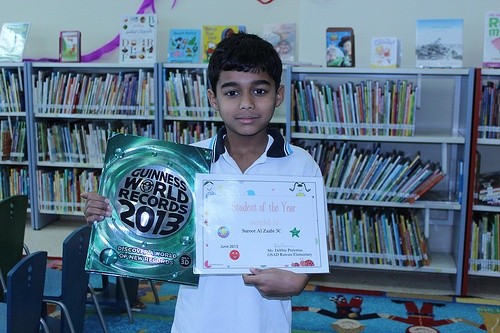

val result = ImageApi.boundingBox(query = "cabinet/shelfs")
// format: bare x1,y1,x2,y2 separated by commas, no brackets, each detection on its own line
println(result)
0,59,500,296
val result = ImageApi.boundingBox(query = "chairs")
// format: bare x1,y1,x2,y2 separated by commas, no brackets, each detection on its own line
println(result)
0,192,160,333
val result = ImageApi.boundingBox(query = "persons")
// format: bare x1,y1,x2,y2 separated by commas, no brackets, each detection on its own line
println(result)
81,31,329,333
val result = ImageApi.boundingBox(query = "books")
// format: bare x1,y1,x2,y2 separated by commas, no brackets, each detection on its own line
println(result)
327,210,430,268
326,27,354,68
0,65,31,210
483,11,500,69
203,25,247,63
119,14,158,65
468,214,500,273
32,70,221,215
477,81,500,139
474,150,500,208
370,36,398,68
416,18,464,69
59,31,81,62
168,28,201,63
291,80,464,208
0,22,31,63
263,22,296,63
